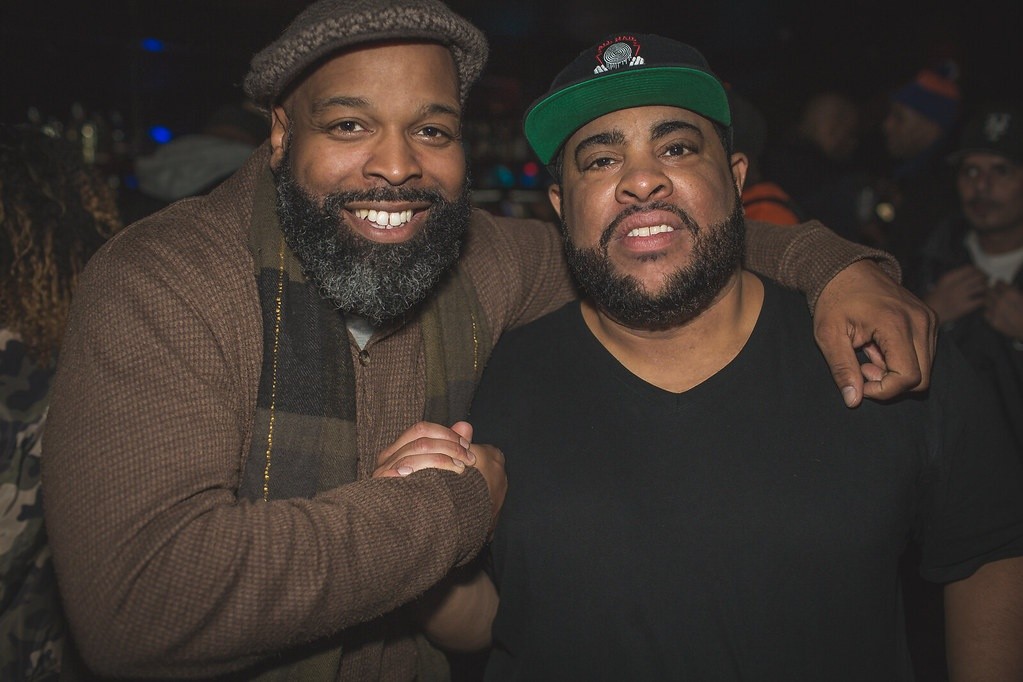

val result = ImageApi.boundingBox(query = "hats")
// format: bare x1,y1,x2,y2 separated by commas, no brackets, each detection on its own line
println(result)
239,0,488,121
943,110,1023,172
888,63,969,134
522,32,733,184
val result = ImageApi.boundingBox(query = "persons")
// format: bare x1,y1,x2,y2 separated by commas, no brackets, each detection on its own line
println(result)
101,54,1022,367
375,29,1023,682
46,0,936,680
